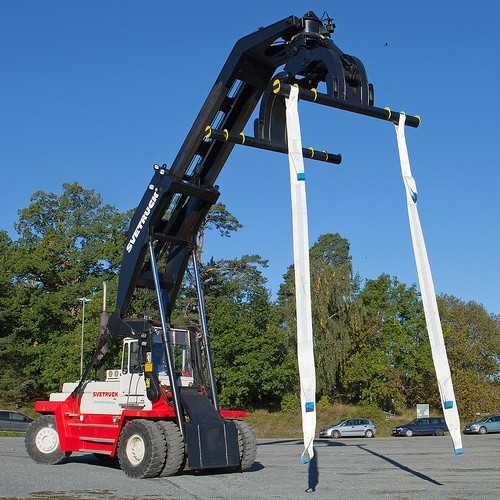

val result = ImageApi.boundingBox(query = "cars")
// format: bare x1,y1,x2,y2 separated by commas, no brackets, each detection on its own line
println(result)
391,417,448,437
319,418,376,438
0,410,35,431
464,414,500,434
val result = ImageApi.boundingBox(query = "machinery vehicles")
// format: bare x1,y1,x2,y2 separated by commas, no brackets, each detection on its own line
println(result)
22,12,462,479
